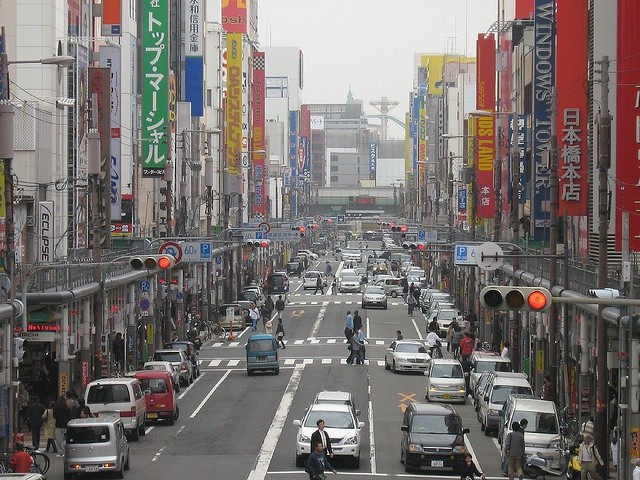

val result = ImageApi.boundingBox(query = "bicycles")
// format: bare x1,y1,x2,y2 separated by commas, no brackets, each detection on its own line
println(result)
432,339,446,358
203,319,226,341
0,444,50,477
0,445,47,474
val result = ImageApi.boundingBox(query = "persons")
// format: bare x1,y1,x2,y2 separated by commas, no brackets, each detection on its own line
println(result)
382,241,385,248
80,409,89,418
452,327,463,359
53,396,70,458
260,305,273,329
297,254,308,278
410,283,415,297
313,275,324,295
541,375,554,400
501,342,510,357
23,396,45,450
346,325,369,365
579,435,604,480
326,261,331,274
475,341,484,351
265,296,274,319
136,361,146,371
408,293,414,317
77,398,93,418
66,390,77,418
459,332,474,360
461,355,476,398
429,316,440,331
172,334,180,341
380,246,382,251
275,318,286,349
465,309,477,326
306,443,337,480
450,317,461,332
311,419,333,458
249,303,260,331
580,417,594,440
283,283,290,303
344,311,354,344
353,310,363,335
331,275,337,295
461,453,483,480
366,242,368,251
359,242,363,252
112,332,125,370
413,288,421,310
397,330,403,340
275,295,285,319
446,323,455,353
504,419,529,480
42,401,57,453
403,282,408,306
568,434,584,480
426,328,443,358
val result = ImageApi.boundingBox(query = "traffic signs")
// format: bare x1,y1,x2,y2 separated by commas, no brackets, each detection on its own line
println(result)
418,230,438,243
259,223,270,234
454,244,487,266
312,214,322,224
158,241,182,267
243,231,263,243
138,280,151,318
182,240,213,262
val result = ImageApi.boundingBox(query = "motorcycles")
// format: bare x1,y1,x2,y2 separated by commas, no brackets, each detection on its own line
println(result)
566,442,582,479
523,447,571,479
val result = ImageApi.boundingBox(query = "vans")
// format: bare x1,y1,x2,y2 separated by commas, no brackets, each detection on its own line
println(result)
479,370,535,437
373,278,408,298
64,417,130,480
245,334,280,376
218,304,246,331
135,371,180,426
503,399,561,471
400,403,470,476
469,354,512,399
84,379,147,441
163,341,200,379
299,250,318,260
424,358,469,406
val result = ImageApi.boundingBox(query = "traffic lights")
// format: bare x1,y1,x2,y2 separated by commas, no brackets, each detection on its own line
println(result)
402,241,428,254
391,226,407,233
291,226,305,231
246,239,271,247
588,288,619,300
307,224,319,229
129,254,175,273
479,286,554,314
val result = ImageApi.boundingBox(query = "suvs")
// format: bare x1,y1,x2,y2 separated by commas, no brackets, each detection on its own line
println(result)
292,404,365,469
152,348,194,387
267,273,289,294
360,286,388,310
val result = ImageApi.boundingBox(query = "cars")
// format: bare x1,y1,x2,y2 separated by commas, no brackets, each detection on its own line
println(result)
233,301,257,326
367,235,429,277
142,361,179,393
474,371,491,411
332,235,347,256
336,251,369,293
313,390,356,414
497,394,541,449
384,339,432,375
240,286,263,311
287,253,308,277
408,277,462,338
309,231,331,256
304,270,328,290
